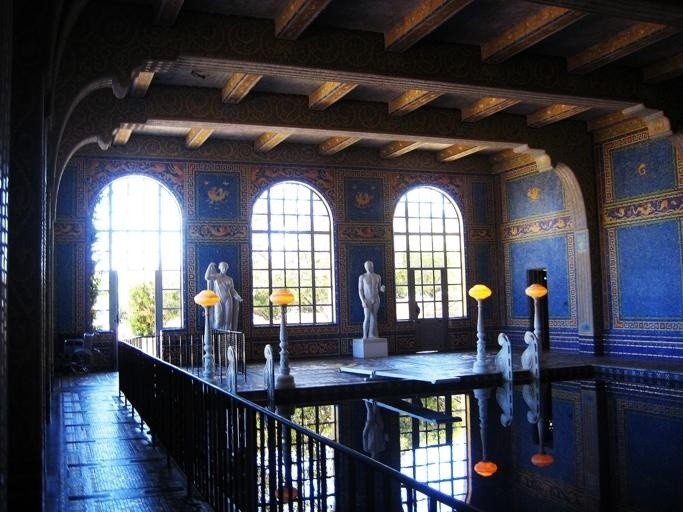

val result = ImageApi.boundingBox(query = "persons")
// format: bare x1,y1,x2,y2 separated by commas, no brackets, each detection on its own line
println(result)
357,261,386,339
361,397,390,466
203,261,244,346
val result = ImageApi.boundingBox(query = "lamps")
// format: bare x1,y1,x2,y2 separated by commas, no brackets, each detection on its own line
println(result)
532,380,556,469
524,283,548,369
473,388,499,477
268,289,296,388
193,289,219,385
467,284,492,374
273,401,299,502
